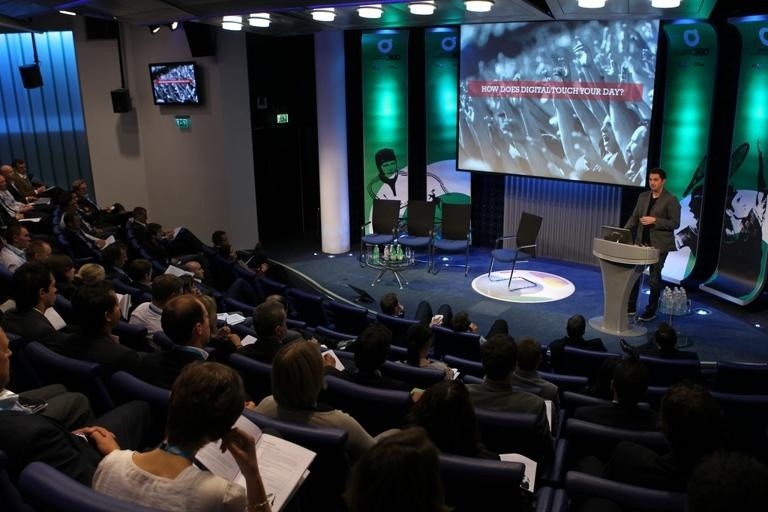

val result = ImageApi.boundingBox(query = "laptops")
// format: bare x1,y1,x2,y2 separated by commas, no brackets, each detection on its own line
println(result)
602,225,633,245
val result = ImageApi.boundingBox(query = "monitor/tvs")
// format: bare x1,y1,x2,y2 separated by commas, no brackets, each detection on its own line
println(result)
149,62,202,106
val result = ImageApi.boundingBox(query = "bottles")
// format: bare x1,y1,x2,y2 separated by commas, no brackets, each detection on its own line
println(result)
660,285,687,314
374,244,402,266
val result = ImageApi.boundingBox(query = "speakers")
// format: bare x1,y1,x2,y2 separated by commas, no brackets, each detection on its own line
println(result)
18,64,42,89
111,88,131,113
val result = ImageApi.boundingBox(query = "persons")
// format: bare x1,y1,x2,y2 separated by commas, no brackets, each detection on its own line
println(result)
722,151,765,280
623,167,682,325
672,183,702,261
148,64,196,101
363,150,408,229
1,155,281,260
1,261,766,510
454,17,660,189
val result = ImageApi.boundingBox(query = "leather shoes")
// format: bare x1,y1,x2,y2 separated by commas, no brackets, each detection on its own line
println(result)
620,339,634,354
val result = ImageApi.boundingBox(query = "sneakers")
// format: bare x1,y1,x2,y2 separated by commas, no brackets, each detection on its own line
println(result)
637,311,656,321
627,308,637,315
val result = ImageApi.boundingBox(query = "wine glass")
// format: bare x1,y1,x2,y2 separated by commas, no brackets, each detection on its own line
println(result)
405,247,415,266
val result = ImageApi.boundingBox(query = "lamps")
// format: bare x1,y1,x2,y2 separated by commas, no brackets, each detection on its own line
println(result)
309,0,495,22
221,13,272,31
147,21,178,35
